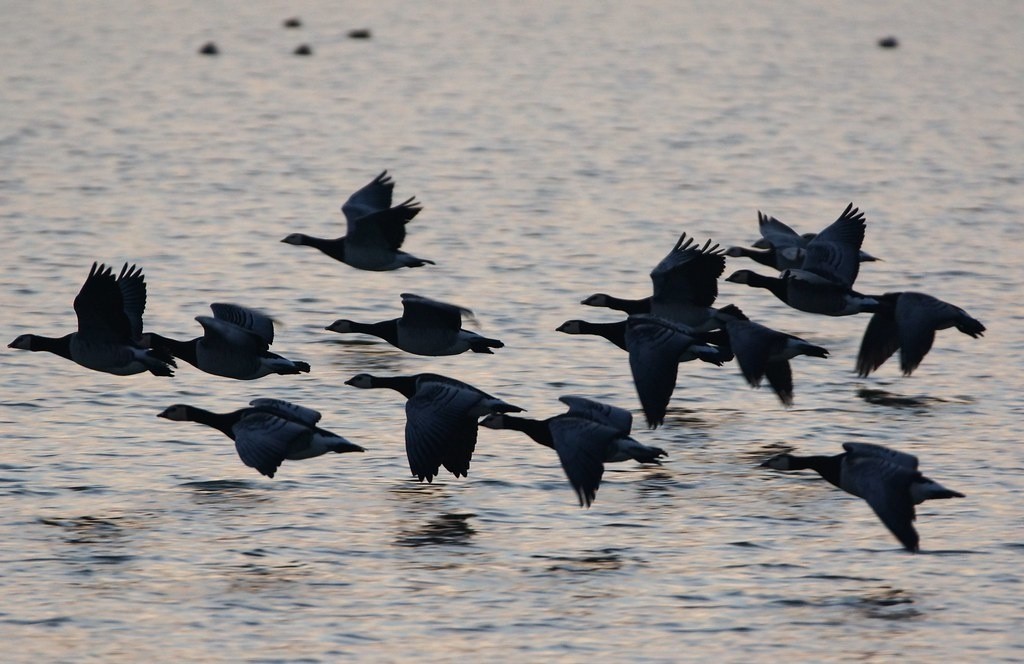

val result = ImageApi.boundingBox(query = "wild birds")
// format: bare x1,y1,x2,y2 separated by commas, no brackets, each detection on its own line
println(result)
477,394,668,512
278,167,437,271
720,200,988,378
156,398,368,479
345,373,529,485
752,442,965,555
7,260,311,380
323,292,505,356
878,34,899,50
555,231,832,434
197,14,373,57
